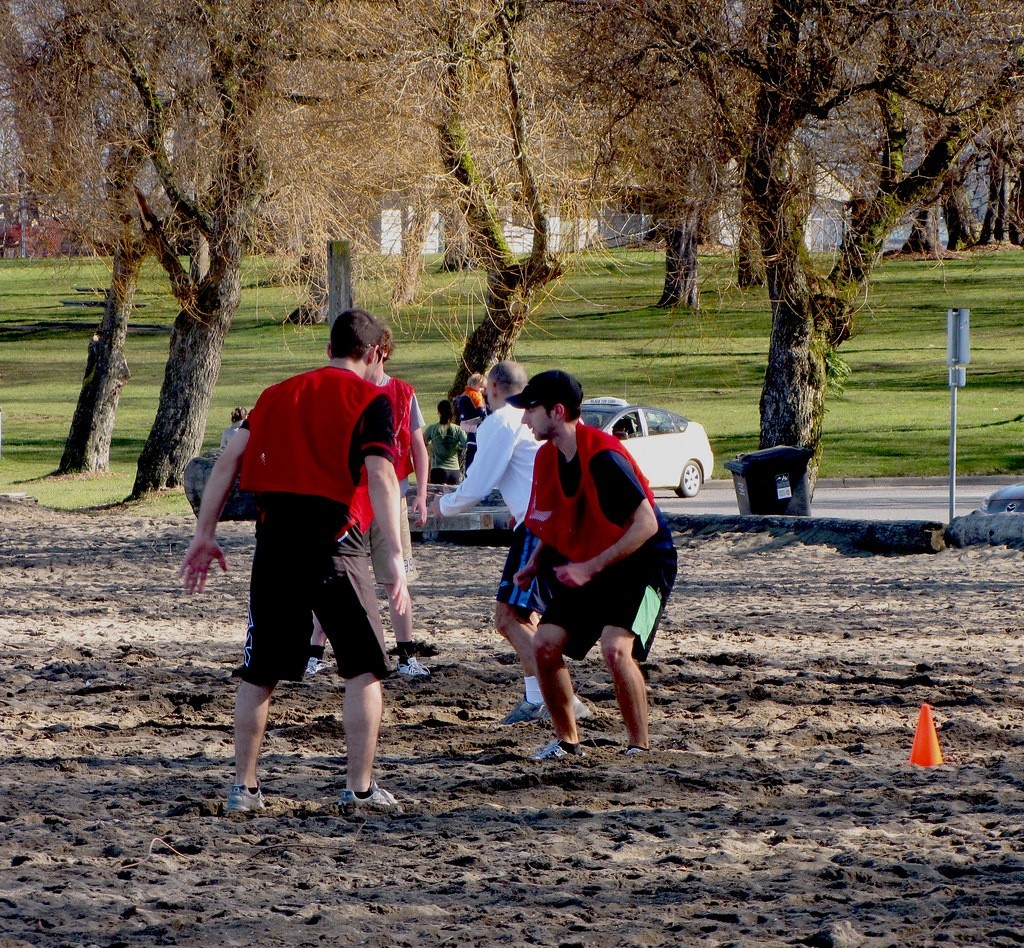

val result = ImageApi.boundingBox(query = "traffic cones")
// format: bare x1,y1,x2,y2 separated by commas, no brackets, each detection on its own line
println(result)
911,703,942,765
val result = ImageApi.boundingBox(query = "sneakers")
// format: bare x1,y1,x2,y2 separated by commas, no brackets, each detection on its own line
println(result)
500,698,551,724
528,737,585,759
305,657,327,674
620,745,652,759
338,779,399,808
226,783,267,816
396,656,430,675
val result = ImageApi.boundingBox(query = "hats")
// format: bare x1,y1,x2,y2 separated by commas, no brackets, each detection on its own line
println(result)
505,368,584,408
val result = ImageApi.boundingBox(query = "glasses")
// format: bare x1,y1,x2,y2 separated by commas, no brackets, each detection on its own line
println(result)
365,343,384,364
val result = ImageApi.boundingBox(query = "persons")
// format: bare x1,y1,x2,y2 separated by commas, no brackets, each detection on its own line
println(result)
423,372,492,485
220,405,248,450
433,361,591,724
505,370,678,760
178,308,408,813
306,319,431,677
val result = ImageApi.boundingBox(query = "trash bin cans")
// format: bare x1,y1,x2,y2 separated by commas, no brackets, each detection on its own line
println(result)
724,445,814,515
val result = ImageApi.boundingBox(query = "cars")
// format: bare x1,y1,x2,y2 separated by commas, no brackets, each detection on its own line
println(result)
581,397,714,498
979,482,1024,514
